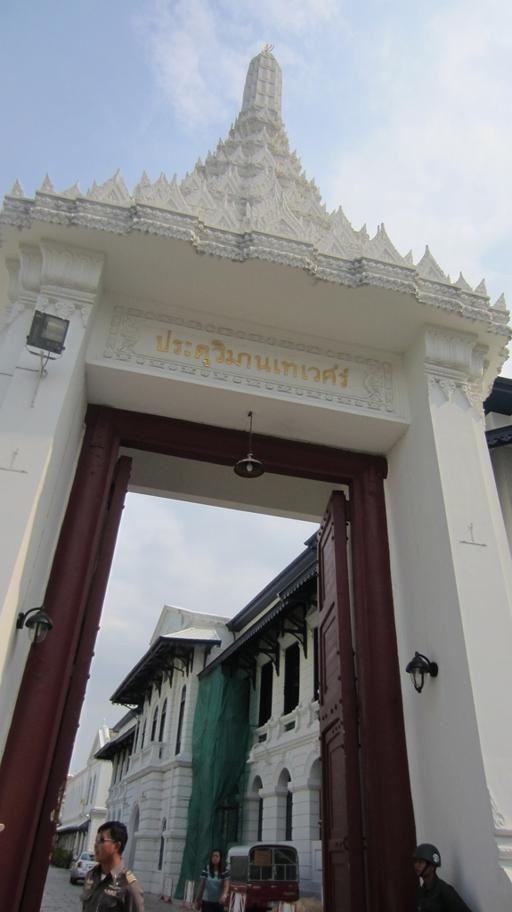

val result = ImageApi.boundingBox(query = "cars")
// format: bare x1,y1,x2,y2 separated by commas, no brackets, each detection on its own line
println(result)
70,851,100,883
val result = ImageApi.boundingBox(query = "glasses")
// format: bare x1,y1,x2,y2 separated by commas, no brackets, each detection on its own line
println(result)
95,834,116,843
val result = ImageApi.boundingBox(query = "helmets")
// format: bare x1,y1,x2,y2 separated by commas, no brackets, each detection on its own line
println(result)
410,842,446,871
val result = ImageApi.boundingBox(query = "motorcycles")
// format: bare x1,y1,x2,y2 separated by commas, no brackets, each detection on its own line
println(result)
221,842,298,912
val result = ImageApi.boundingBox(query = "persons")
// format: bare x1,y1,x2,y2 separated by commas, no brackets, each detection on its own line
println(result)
409,843,474,911
78,821,145,911
192,849,231,910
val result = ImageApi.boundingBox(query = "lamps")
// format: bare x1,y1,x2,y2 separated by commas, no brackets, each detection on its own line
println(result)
234,411,265,477
16,605,53,646
26,310,69,354
405,651,438,693
